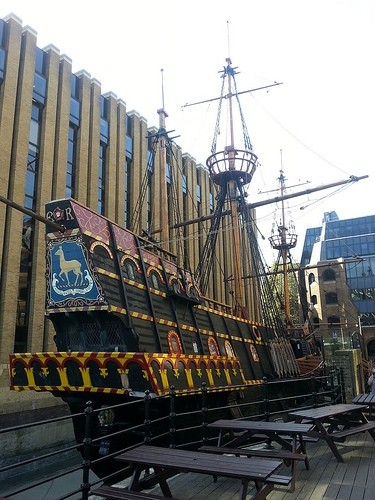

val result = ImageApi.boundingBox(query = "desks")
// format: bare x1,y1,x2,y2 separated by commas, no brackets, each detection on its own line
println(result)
113,444,283,500
206,419,314,492
288,404,368,463
352,394,375,421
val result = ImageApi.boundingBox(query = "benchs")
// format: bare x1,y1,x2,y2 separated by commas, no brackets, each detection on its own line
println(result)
326,422,375,443
224,432,319,443
90,485,174,500
282,418,314,424
263,474,293,486
197,446,307,461
